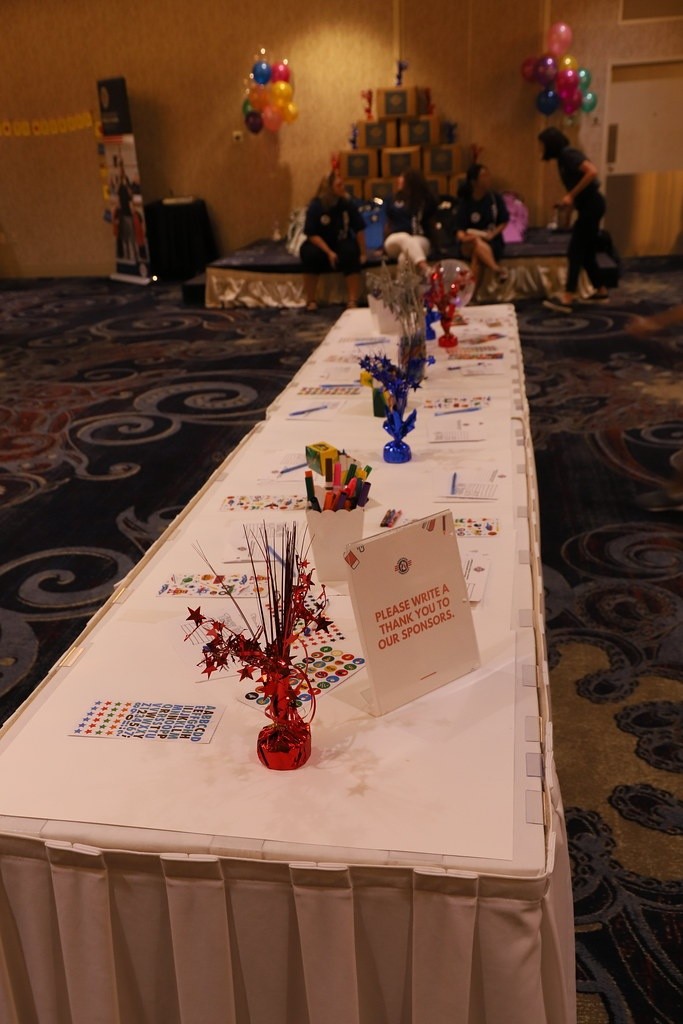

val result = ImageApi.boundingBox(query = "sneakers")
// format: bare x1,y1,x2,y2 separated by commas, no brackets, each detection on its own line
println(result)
542,296,572,313
578,293,609,304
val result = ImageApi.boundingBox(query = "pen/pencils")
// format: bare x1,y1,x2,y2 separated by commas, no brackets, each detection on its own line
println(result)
355,339,390,347
310,496,321,514
447,362,483,371
434,406,481,417
303,469,315,509
323,457,372,511
320,384,363,388
289,406,328,416
451,473,456,495
279,462,307,475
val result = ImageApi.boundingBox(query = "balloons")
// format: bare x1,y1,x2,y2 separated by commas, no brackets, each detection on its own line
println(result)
522,54,598,126
548,23,572,55
242,60,299,135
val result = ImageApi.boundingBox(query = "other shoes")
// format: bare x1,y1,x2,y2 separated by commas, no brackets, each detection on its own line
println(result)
494,266,508,285
305,300,317,311
346,299,357,308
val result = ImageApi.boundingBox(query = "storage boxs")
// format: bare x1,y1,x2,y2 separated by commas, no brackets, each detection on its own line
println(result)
337,85,467,200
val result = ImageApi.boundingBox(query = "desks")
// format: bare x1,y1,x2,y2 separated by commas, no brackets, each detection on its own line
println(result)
265,302,531,417
0,418,577,1024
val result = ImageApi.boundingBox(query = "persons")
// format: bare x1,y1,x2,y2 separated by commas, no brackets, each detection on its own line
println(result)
300,174,368,311
538,127,610,312
453,164,510,283
375,171,432,278
112,158,139,261
